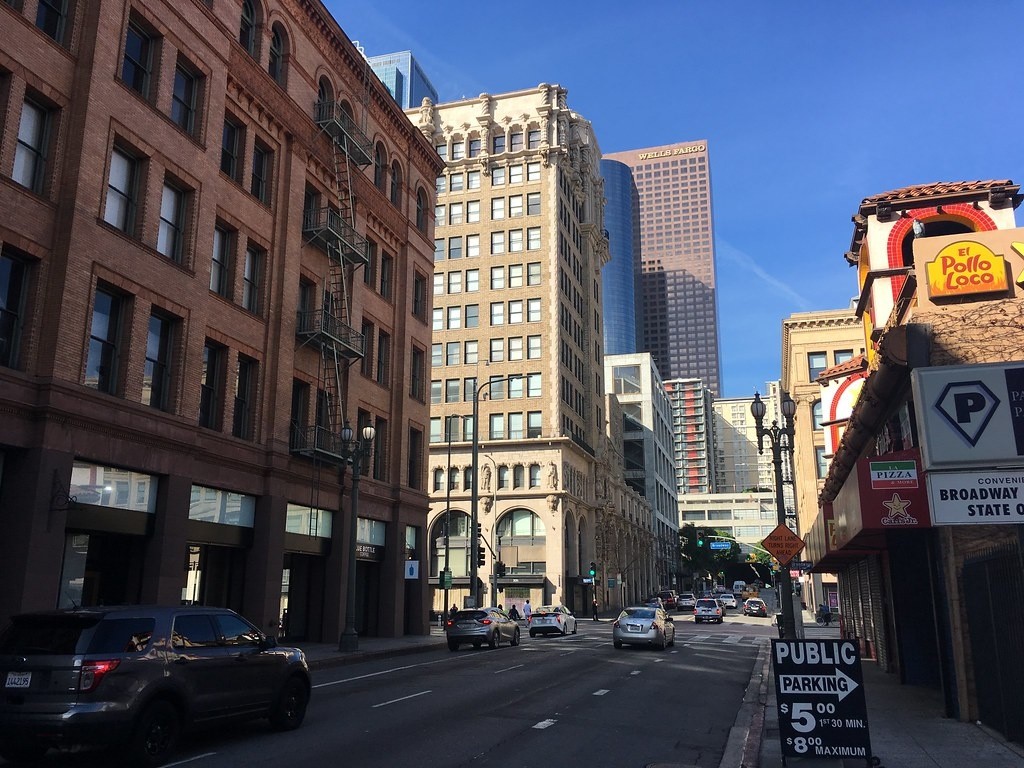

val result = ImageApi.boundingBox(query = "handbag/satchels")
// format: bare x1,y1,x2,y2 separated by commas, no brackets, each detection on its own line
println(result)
508,613,511,617
528,616,532,622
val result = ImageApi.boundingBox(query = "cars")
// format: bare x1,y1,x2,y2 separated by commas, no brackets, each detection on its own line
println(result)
676,594,697,612
718,594,738,609
743,598,768,617
642,589,679,612
613,606,676,651
694,599,724,624
528,605,578,638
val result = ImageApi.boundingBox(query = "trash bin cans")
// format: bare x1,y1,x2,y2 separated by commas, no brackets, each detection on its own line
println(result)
776,614,785,638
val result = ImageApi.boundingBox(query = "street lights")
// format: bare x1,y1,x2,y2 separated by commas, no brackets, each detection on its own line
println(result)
485,455,498,608
335,418,376,652
443,413,470,632
751,388,797,639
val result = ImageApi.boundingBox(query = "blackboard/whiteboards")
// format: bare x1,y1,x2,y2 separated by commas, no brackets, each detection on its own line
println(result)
770,639,873,757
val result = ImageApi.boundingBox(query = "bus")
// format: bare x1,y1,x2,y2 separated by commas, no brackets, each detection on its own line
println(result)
699,580,762,602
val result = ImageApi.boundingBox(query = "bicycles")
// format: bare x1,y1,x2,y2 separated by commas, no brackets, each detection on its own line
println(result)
815,611,840,627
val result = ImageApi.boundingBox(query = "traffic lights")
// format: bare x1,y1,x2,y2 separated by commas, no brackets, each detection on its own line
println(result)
747,555,750,561
478,523,483,539
478,545,486,568
696,530,705,547
589,561,595,576
499,563,506,578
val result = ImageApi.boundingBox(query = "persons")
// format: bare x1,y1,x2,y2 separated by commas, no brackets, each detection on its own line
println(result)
498,600,535,628
450,604,458,618
819,603,830,626
591,598,600,621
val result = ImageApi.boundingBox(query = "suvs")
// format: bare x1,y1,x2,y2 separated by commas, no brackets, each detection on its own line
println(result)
0,601,313,768
445,607,521,653
